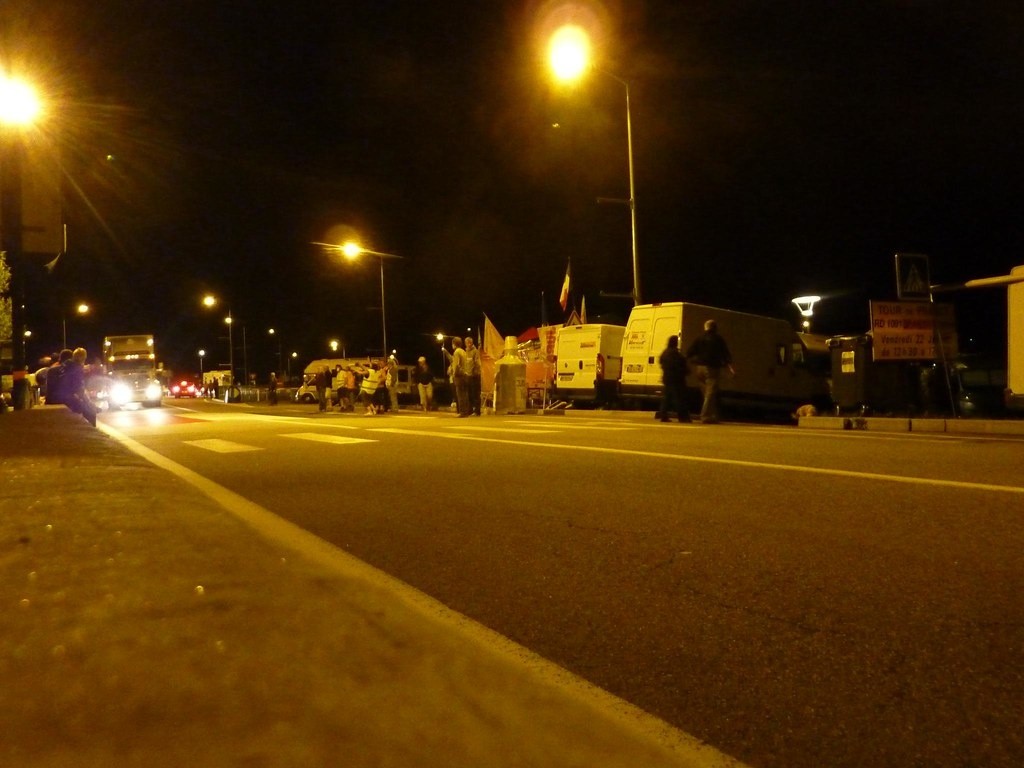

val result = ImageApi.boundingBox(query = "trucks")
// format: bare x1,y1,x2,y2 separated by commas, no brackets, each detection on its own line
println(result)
103,334,164,412
615,302,831,418
295,353,419,403
552,324,628,409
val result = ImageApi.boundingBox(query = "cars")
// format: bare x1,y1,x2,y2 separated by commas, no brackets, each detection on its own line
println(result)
174,380,195,399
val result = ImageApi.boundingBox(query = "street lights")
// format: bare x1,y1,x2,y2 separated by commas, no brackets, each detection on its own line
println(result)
287,352,298,387
547,22,640,308
62,304,89,349
223,310,233,371
343,237,387,359
201,294,234,402
0,73,41,412
436,332,446,379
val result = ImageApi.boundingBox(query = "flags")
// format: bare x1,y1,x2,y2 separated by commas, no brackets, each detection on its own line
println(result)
483,317,505,361
559,262,571,312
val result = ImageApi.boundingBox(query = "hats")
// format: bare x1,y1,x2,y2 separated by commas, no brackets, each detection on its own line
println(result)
419,357,426,361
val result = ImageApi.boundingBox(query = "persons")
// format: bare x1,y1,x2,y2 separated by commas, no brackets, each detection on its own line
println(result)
441,335,483,418
687,319,737,423
314,358,399,416
268,373,278,406
204,377,220,399
414,357,439,411
35,348,97,427
659,335,693,423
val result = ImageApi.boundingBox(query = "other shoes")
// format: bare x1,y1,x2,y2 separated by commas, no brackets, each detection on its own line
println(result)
373,411,376,414
364,412,372,415
661,418,672,422
